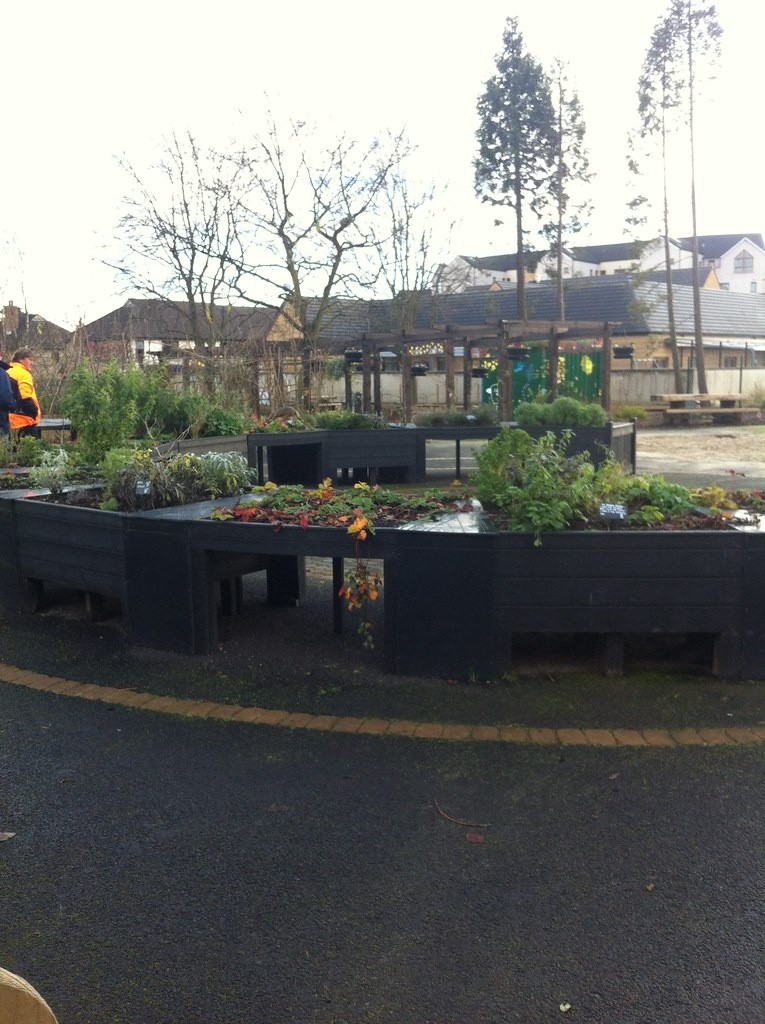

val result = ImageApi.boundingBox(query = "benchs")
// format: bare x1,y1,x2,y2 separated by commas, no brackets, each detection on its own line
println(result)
664,407,761,415
646,407,667,413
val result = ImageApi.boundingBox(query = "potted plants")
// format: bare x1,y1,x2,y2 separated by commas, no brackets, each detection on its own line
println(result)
0,400,765,671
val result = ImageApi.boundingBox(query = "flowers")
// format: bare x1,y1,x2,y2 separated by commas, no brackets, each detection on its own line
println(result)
211,479,384,650
693,467,765,531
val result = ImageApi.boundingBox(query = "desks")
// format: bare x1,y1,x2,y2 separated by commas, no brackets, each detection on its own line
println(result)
649,392,749,427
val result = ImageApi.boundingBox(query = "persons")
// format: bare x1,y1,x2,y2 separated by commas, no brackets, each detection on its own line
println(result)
4,347,41,442
0,352,23,447
